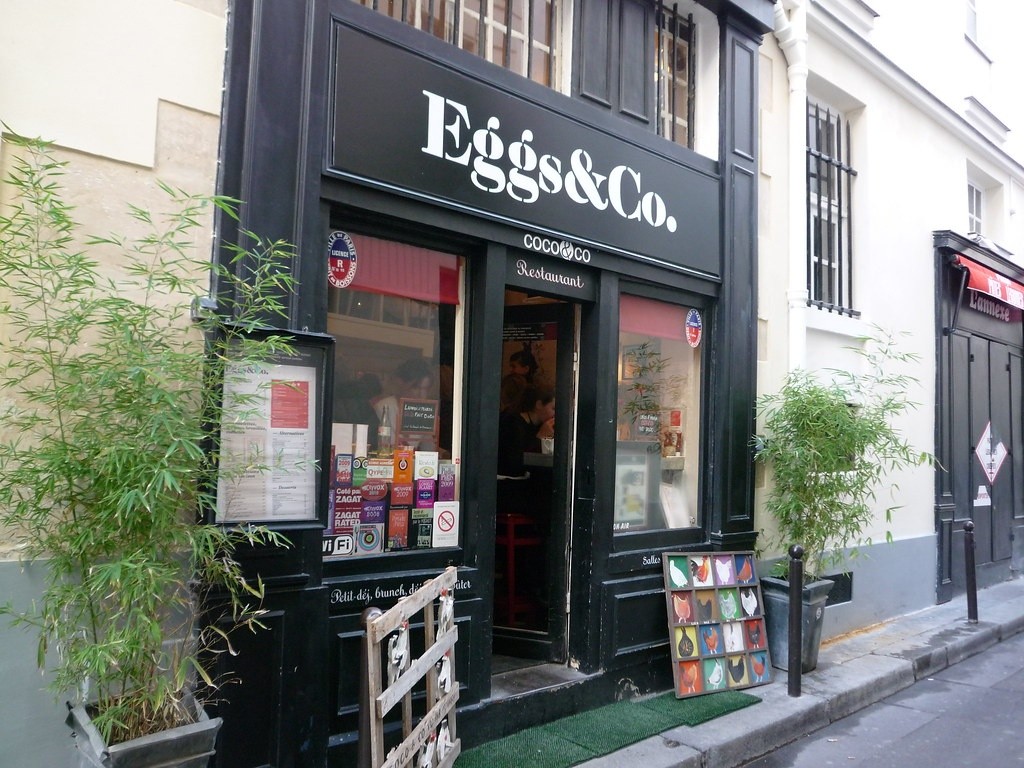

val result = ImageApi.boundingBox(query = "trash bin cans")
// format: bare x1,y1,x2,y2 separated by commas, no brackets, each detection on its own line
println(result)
759,569,835,674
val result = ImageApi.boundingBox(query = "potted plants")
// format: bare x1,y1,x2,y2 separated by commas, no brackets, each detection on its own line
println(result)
0,123,325,767
748,321,949,673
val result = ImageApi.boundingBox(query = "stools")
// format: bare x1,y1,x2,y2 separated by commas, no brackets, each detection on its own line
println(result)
495,511,536,623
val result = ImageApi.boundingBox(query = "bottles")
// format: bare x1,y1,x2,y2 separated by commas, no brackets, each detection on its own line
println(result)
377,405,391,459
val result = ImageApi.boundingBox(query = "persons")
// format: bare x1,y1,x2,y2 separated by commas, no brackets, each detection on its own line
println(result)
496,352,556,597
334,357,453,446
